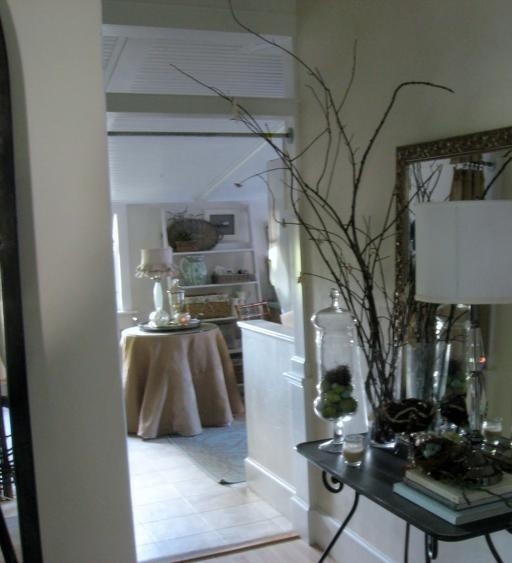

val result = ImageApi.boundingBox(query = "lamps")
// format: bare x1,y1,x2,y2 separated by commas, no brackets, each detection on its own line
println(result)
410,195,512,486
133,245,178,327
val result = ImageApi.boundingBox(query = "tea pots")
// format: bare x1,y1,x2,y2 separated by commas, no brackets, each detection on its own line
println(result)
178,253,209,285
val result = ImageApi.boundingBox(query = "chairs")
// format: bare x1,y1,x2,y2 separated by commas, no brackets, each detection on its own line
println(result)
230,298,274,325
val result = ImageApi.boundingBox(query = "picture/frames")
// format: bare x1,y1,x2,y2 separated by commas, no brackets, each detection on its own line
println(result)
202,207,241,243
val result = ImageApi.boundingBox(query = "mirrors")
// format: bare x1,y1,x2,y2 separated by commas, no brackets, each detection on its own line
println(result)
385,124,511,471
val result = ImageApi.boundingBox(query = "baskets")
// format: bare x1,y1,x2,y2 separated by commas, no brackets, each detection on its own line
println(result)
183,290,233,319
211,271,250,283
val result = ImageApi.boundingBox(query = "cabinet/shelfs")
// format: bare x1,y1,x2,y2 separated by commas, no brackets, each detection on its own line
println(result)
154,201,264,359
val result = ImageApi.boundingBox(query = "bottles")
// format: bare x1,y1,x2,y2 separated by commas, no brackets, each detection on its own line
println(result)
166,277,185,326
310,285,361,453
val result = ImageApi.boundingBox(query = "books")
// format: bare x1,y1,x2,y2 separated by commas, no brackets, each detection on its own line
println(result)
402,476,511,512
404,467,512,505
392,481,511,527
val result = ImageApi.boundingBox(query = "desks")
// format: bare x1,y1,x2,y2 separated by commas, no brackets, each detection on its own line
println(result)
293,427,512,561
119,322,247,441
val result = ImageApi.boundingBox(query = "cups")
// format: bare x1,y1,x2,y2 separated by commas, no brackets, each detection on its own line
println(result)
341,432,366,470
174,310,192,327
479,415,503,446
